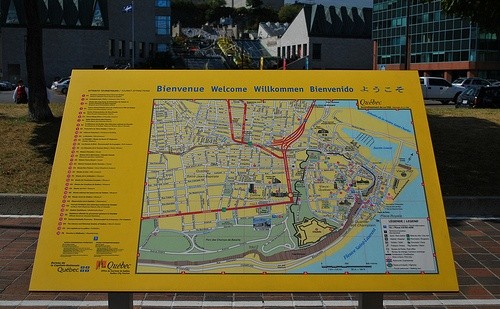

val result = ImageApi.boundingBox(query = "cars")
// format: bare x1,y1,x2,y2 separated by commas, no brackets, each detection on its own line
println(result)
55,79,70,93
52,76,71,90
455,86,500,109
452,77,499,88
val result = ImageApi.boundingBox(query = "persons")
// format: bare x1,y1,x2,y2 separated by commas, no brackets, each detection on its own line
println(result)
170,57,190,70
12,79,29,105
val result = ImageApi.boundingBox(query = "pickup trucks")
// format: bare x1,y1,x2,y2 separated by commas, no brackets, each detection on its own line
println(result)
420,76,466,104
